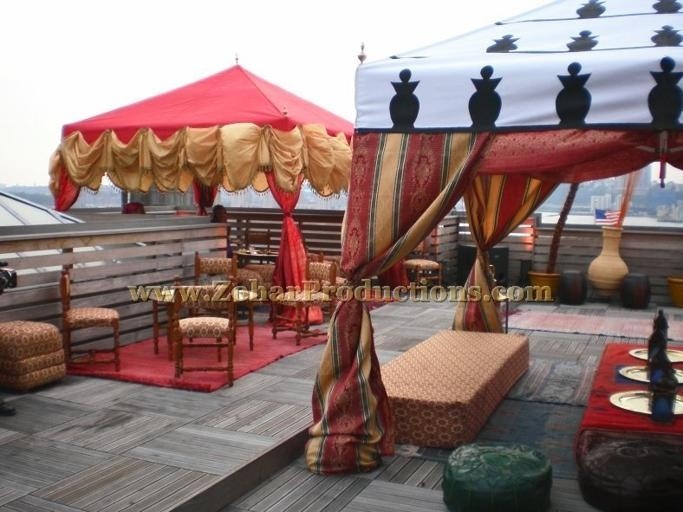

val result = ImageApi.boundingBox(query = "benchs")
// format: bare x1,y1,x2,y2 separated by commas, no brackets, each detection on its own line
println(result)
380,331,529,450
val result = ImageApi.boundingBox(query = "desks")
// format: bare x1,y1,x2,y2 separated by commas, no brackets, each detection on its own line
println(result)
575,342,683,468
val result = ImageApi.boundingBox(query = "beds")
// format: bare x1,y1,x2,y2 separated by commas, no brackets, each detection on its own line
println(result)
150,286,258,362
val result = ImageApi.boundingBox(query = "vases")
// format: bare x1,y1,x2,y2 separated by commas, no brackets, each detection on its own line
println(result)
587,226,628,297
668,276,683,308
528,270,559,299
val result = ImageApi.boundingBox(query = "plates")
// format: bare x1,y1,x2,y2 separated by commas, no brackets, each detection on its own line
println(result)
606,348,683,417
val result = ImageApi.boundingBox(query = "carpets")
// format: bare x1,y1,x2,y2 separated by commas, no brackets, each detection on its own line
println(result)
499,308,683,343
67,322,328,392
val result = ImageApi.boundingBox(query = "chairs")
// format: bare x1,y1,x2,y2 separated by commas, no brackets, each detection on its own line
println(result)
273,257,337,347
172,275,235,388
404,241,443,290
187,250,238,344
245,228,271,248
59,265,121,373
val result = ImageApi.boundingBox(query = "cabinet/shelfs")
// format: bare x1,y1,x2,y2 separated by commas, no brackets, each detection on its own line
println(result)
456,243,508,287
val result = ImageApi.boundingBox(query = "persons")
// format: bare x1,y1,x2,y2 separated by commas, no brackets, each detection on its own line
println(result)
0,261,17,416
210,204,233,258
121,202,145,214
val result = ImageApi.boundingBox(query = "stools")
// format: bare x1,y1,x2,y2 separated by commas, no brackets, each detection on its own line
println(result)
621,275,652,309
0,321,67,393
441,442,552,512
559,270,586,304
580,440,683,512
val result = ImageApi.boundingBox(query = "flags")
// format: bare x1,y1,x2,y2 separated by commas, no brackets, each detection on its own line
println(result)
595,209,621,226
